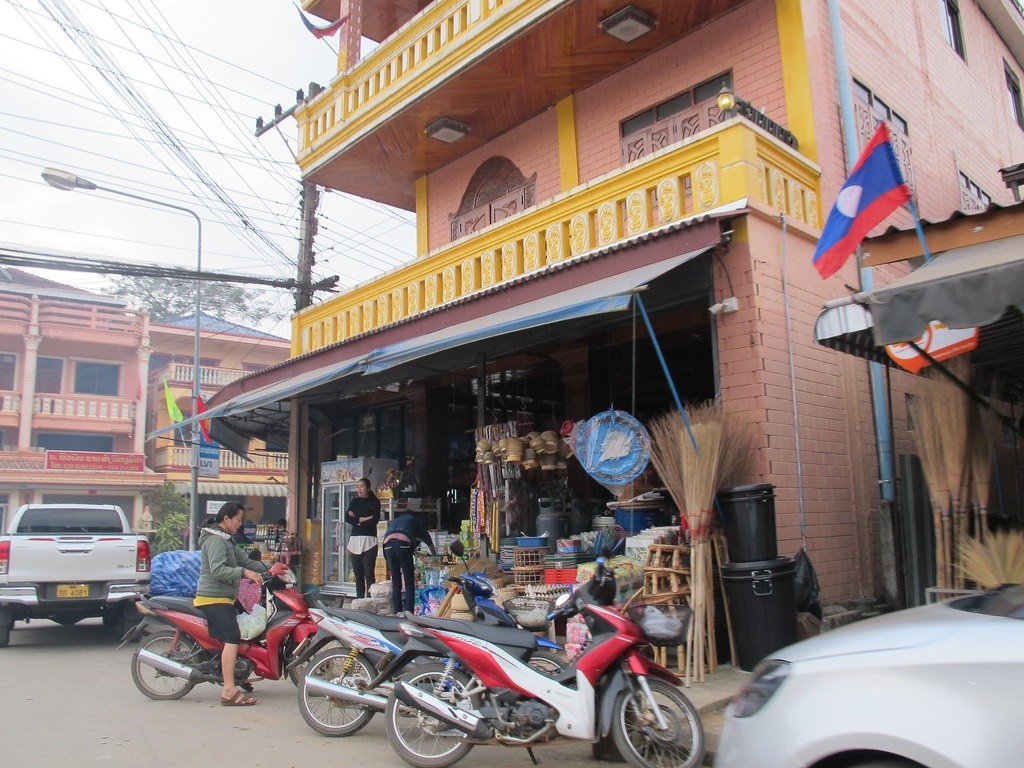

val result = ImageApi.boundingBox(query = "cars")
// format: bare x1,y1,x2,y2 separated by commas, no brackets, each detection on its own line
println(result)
712,578,1024,768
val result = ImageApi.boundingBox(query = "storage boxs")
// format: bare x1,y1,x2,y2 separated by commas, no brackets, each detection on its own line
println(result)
372,488,417,582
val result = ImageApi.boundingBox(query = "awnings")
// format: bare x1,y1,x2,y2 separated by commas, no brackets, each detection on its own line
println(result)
852,234,1024,347
146,246,736,520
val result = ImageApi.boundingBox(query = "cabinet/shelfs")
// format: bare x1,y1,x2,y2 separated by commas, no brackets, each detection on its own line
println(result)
511,545,550,593
379,497,441,542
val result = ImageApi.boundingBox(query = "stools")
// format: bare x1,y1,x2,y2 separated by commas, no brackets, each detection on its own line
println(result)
641,543,693,675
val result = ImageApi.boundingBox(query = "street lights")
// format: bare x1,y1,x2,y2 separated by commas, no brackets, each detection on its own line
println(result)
40,166,202,552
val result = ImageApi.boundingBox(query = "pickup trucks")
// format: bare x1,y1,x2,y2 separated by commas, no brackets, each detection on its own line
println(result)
0,503,152,647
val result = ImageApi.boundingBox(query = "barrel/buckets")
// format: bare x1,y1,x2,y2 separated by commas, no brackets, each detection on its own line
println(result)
715,483,776,562
592,516,615,549
613,508,660,531
720,556,797,672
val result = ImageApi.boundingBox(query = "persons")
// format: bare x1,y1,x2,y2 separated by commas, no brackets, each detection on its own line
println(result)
382,509,437,615
345,478,381,598
193,502,273,705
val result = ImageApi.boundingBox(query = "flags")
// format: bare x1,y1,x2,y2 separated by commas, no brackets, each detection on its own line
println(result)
191,380,213,444
164,379,183,421
813,124,910,280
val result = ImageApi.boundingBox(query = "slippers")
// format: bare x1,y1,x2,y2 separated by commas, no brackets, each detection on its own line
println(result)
241,683,254,692
221,690,257,706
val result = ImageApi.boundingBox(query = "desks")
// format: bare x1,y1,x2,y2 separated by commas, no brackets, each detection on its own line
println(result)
264,551,301,593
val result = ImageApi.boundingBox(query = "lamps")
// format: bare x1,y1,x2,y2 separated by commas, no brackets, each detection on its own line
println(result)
715,81,735,121
599,5,658,43
424,118,471,144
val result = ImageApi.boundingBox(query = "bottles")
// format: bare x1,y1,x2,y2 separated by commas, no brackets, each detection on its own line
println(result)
350,496,357,503
256,524,282,552
413,554,441,571
238,543,255,553
335,463,348,481
414,569,447,584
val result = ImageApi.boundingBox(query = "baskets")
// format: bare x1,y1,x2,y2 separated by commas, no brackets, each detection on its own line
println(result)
303,593,347,609
627,604,692,646
502,597,555,631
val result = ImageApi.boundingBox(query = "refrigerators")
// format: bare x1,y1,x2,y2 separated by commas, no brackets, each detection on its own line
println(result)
319,458,401,597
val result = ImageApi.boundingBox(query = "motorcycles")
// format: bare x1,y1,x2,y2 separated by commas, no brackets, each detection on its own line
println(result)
284,539,577,746
363,531,709,768
115,549,357,698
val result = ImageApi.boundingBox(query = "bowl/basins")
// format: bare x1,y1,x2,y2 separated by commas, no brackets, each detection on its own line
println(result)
516,537,548,547
